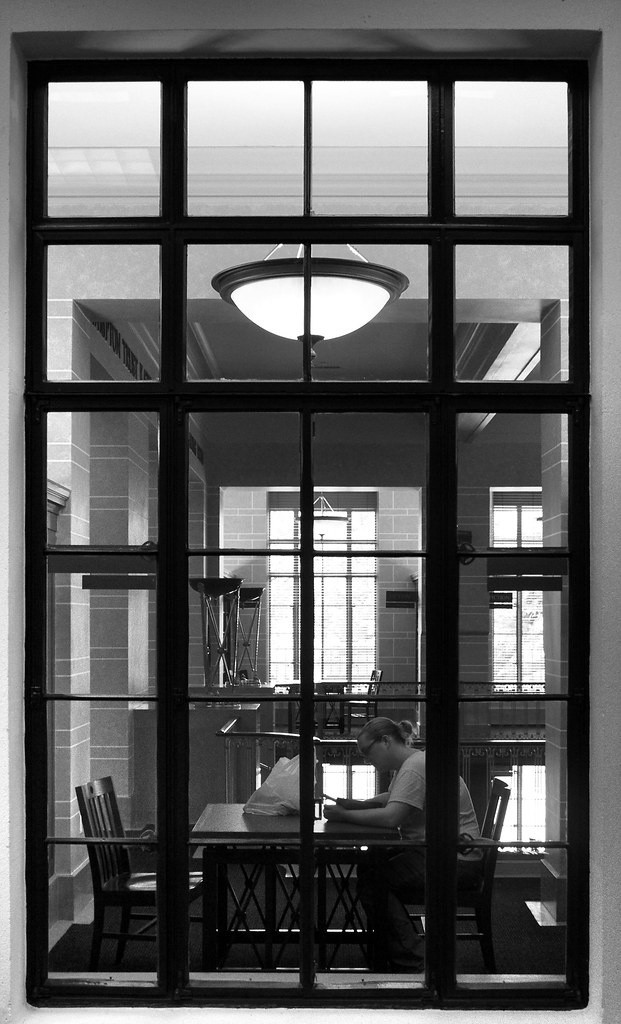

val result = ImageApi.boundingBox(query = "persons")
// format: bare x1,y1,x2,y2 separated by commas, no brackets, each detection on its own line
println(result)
323,717,483,973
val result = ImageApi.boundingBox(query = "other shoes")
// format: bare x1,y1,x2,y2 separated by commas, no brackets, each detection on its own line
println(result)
372,959,424,974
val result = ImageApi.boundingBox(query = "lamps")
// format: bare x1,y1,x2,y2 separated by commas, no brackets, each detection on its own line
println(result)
210,210,409,368
295,486,348,537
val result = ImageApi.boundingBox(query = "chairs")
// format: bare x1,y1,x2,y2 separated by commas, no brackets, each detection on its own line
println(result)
75,776,203,973
394,779,511,974
340,670,383,723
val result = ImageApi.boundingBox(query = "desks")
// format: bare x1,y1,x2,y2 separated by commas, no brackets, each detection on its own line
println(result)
276,682,349,733
188,803,401,972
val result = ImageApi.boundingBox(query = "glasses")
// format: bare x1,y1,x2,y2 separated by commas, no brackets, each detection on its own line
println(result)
360,738,381,759
